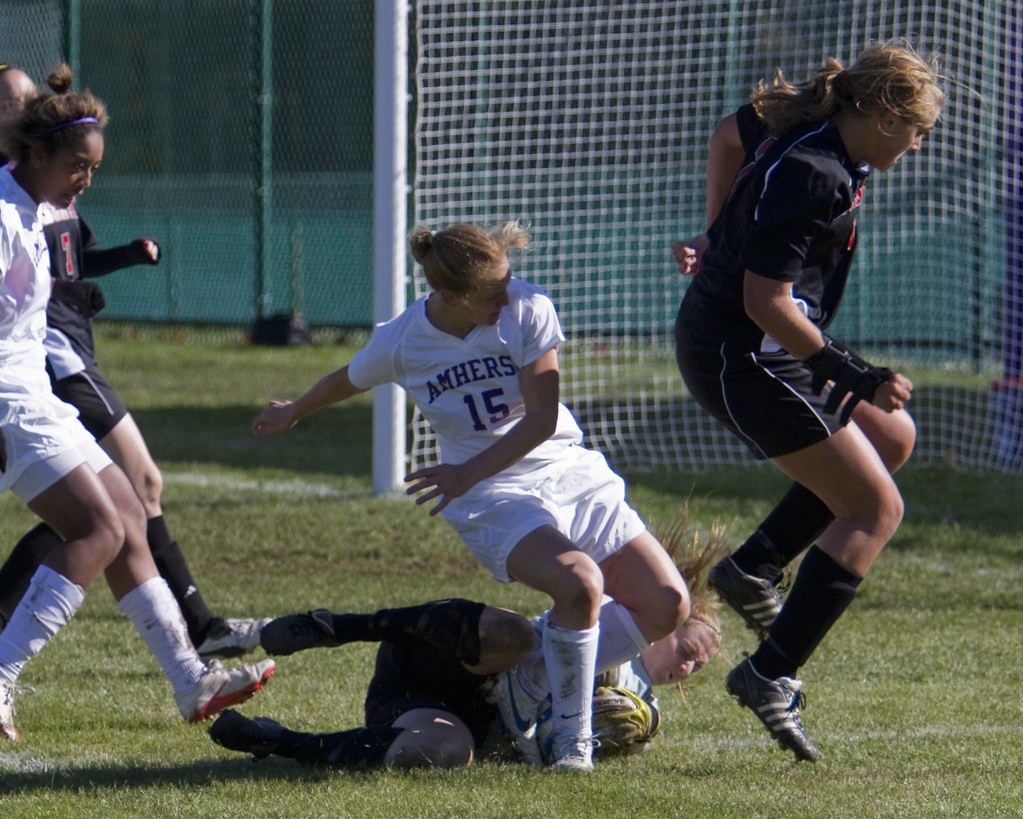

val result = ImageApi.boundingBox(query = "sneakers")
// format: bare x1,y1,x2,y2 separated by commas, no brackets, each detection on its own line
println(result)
706,555,791,638
0,679,36,742
725,656,823,764
173,658,277,722
493,662,543,768
546,734,602,771
196,617,273,660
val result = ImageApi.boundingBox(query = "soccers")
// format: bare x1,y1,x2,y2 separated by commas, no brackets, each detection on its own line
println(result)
536,677,597,766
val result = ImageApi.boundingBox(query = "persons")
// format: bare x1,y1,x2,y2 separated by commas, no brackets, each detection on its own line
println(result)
0,65,275,658
207,519,726,765
673,47,942,762
0,64,274,744
254,224,692,771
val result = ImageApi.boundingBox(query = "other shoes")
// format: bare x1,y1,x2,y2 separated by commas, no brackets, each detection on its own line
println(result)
208,710,274,762
260,609,332,655
